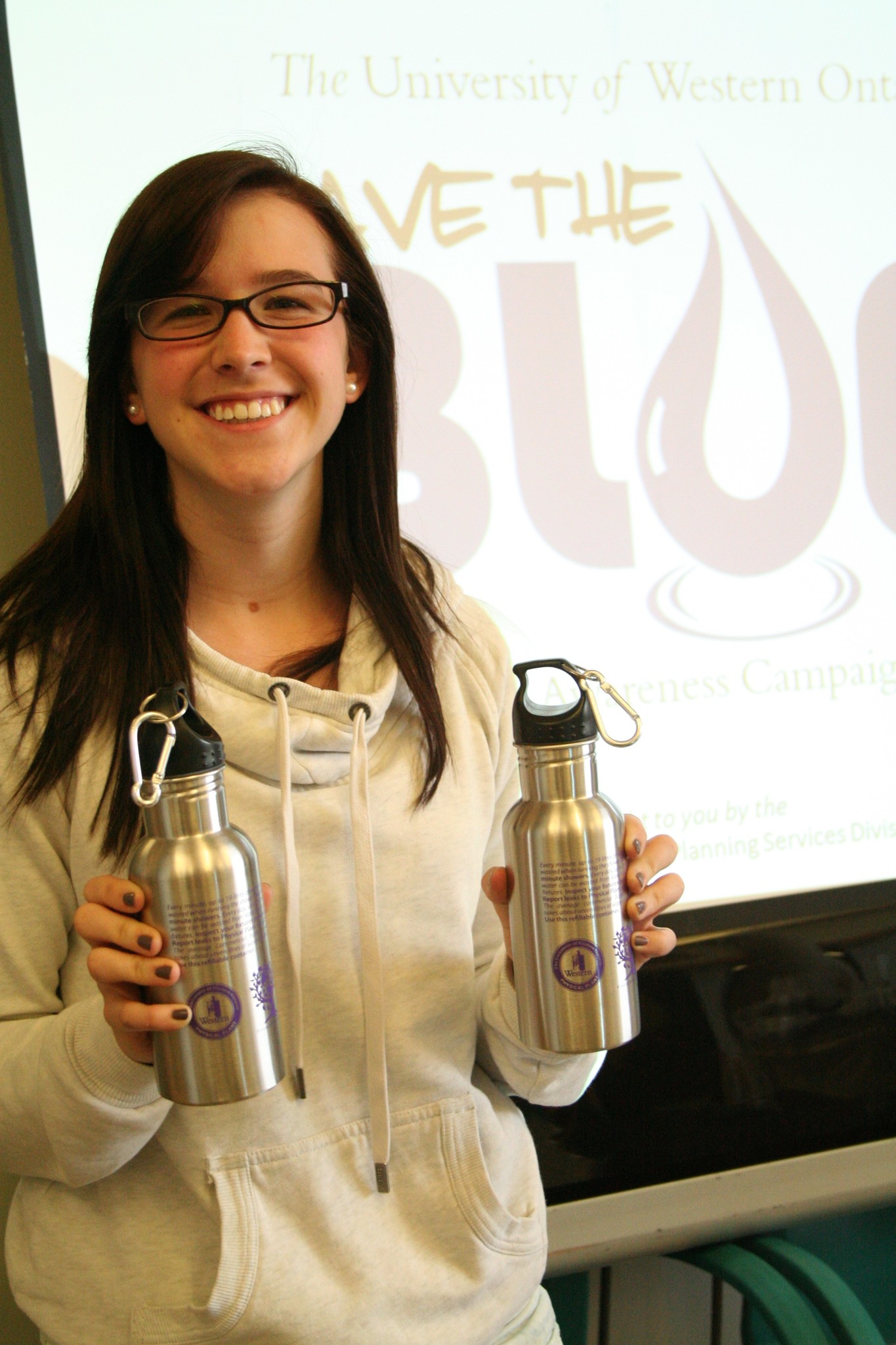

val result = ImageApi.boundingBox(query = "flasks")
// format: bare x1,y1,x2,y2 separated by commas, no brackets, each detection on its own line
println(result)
130,678,283,1107
502,659,645,1055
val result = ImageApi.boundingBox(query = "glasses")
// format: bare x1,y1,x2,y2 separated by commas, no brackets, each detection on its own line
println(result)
124,281,352,341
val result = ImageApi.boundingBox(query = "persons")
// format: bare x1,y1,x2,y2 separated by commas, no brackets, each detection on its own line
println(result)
0,147,686,1345
